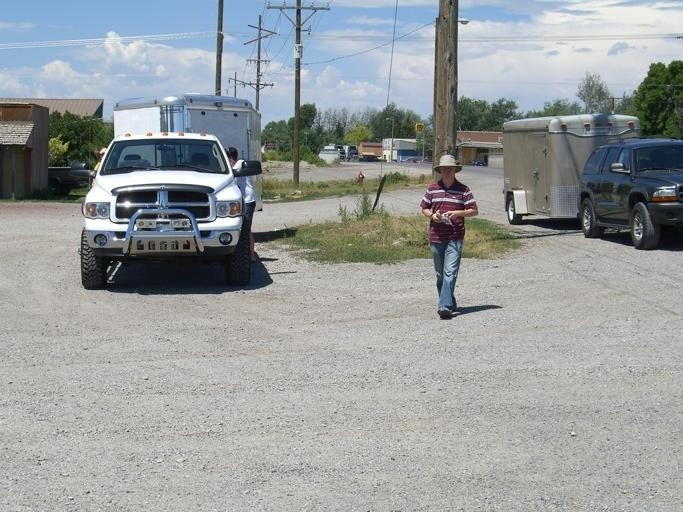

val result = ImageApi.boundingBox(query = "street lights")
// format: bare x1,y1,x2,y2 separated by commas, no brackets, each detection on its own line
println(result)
432,17,469,178
386,118,394,161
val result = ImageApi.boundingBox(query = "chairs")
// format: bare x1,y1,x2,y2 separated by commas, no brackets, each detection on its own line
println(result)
192,154,210,169
122,155,143,170
639,159,651,169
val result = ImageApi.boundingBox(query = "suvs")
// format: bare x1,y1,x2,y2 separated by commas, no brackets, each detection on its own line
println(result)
340,149,345,159
579,138,682,249
80,131,260,291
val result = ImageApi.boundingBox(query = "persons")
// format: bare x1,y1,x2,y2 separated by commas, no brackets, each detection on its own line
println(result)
224,145,257,263
418,155,478,319
93,147,106,177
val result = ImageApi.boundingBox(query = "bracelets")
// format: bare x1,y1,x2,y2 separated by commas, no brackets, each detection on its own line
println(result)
429,212,433,221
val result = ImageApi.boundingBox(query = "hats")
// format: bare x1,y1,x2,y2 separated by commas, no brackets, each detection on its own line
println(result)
433,155,463,174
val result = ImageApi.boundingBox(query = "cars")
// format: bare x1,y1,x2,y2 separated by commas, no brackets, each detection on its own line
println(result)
472,160,486,166
401,157,418,164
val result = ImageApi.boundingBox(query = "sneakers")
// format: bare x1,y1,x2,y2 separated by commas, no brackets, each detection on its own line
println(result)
438,306,452,319
